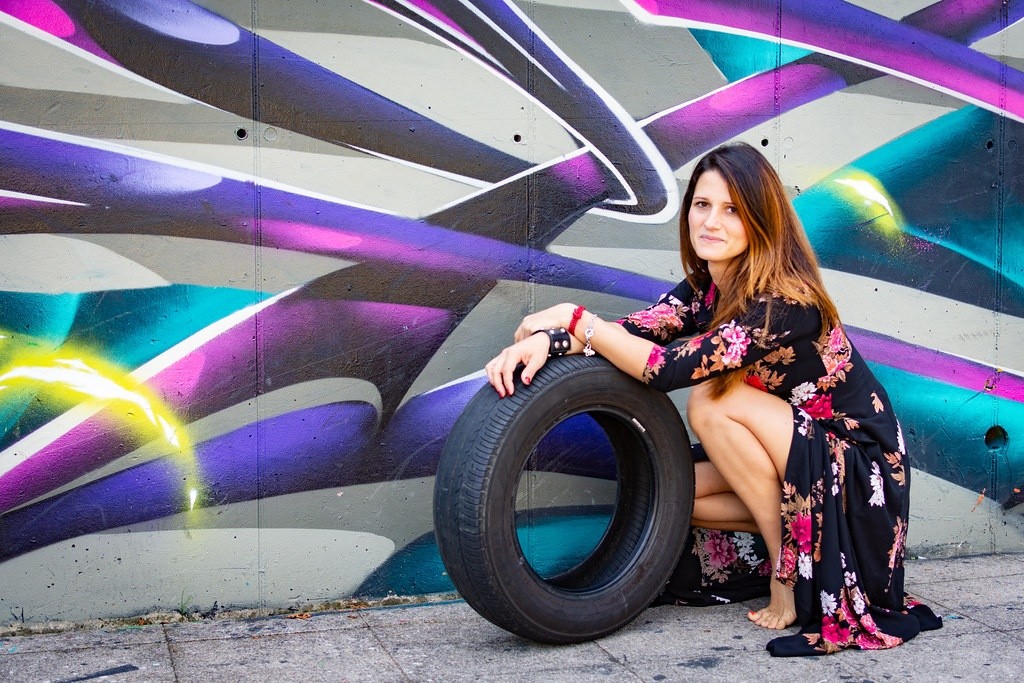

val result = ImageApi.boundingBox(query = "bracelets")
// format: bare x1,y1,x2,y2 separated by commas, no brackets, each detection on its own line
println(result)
568,307,586,335
532,327,571,357
583,314,597,356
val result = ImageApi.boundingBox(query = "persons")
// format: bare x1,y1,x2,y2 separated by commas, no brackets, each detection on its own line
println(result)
485,141,943,657
